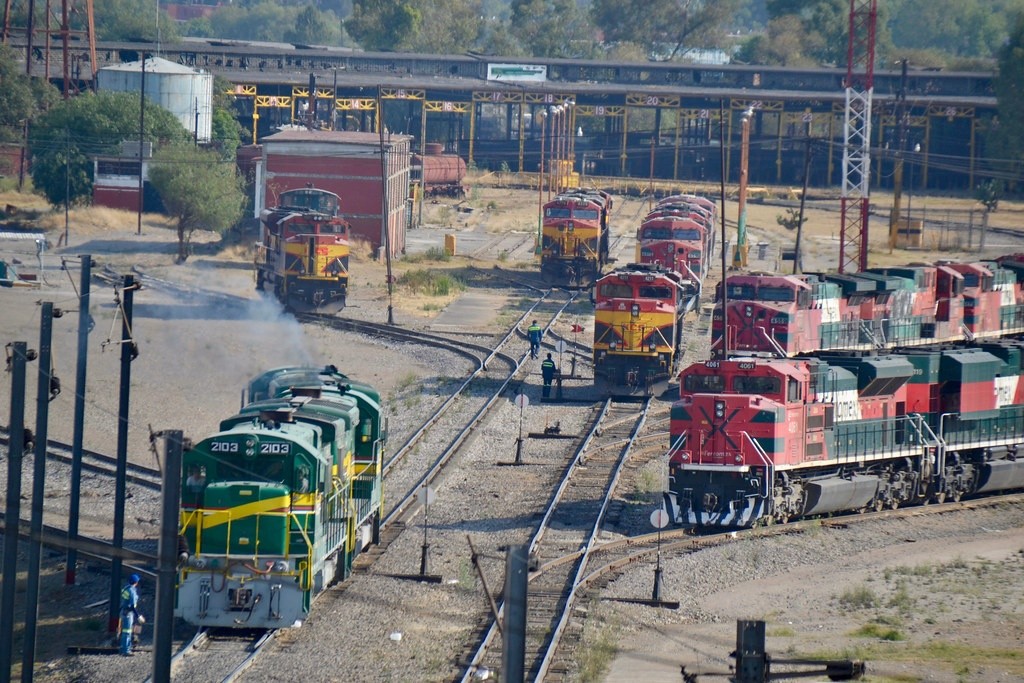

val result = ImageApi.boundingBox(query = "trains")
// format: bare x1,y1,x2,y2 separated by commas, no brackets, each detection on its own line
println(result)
254,184,353,319
710,251,1024,359
589,194,720,383
664,334,1024,532
181,364,392,627
408,143,474,198
541,186,614,282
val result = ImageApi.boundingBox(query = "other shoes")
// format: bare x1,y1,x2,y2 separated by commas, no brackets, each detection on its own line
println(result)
534,354,539,359
119,651,135,656
531,355,533,360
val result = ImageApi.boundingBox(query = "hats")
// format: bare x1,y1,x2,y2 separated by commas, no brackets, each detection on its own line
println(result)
128,574,140,584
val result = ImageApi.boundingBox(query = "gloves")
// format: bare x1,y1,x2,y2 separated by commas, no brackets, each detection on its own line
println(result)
137,614,146,624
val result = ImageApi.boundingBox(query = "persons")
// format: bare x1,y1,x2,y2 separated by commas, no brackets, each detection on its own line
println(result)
540,352,557,397
527,320,542,359
120,573,145,656
